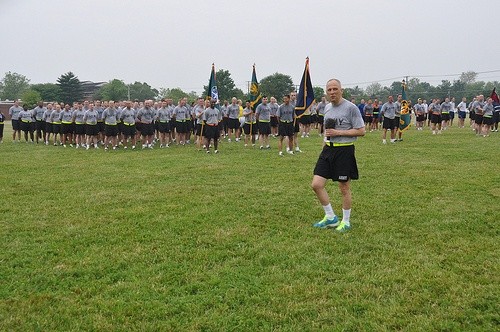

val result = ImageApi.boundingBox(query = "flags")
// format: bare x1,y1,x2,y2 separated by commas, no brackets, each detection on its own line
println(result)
207,65,220,102
250,66,263,113
293,59,315,118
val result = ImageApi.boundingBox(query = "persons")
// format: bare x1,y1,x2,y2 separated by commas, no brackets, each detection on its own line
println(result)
312,79,367,231
0,87,500,156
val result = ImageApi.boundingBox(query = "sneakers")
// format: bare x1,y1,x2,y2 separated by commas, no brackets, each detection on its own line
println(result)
1,122,500,156
314,215,340,228
335,222,351,232
